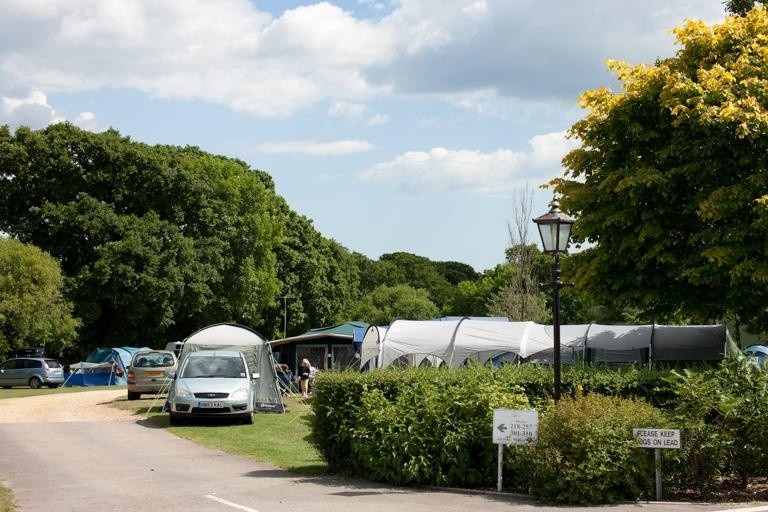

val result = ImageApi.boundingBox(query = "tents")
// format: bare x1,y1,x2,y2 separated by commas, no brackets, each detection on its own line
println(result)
85,346,153,385
161,322,285,413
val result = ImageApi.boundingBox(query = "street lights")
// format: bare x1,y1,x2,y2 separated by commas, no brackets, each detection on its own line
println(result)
531,195,578,405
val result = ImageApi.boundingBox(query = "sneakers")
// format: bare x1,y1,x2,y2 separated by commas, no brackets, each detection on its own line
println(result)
302,395,307,397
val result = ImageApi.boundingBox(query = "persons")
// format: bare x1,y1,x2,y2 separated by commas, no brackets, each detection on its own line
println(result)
300,358,310,396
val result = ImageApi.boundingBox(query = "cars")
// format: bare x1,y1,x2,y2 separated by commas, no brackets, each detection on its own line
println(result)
0,358,65,388
127,350,260,425
747,344,768,365
